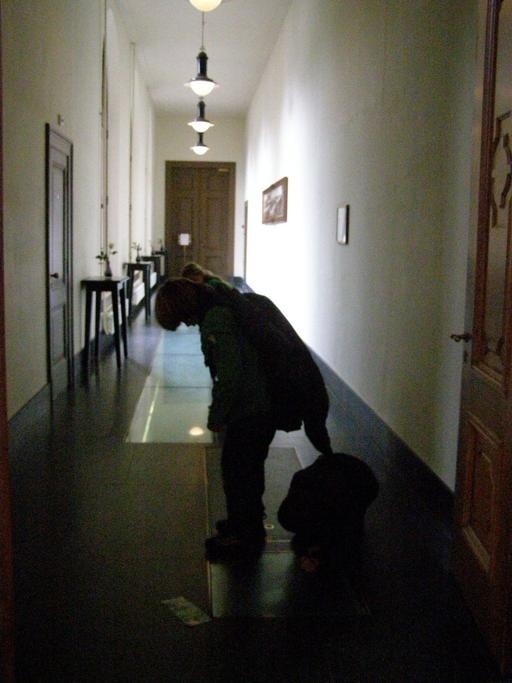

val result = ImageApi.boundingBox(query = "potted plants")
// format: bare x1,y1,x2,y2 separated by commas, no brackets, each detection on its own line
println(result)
95,244,118,277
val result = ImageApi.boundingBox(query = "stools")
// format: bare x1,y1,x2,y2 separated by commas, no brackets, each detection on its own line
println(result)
80,251,168,369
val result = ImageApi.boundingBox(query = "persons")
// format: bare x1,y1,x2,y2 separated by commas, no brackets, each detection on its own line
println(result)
277,450,380,574
180,260,230,294
154,277,283,555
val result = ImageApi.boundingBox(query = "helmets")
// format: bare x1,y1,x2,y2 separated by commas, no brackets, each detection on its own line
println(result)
155,262,202,332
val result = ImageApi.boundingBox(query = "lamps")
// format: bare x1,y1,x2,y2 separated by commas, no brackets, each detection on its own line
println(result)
184,0,223,157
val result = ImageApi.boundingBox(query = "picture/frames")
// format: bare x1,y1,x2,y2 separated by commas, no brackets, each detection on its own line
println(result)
336,203,350,245
261,176,288,224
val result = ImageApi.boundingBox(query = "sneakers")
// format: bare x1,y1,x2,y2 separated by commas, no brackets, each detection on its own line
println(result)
204,518,266,569
